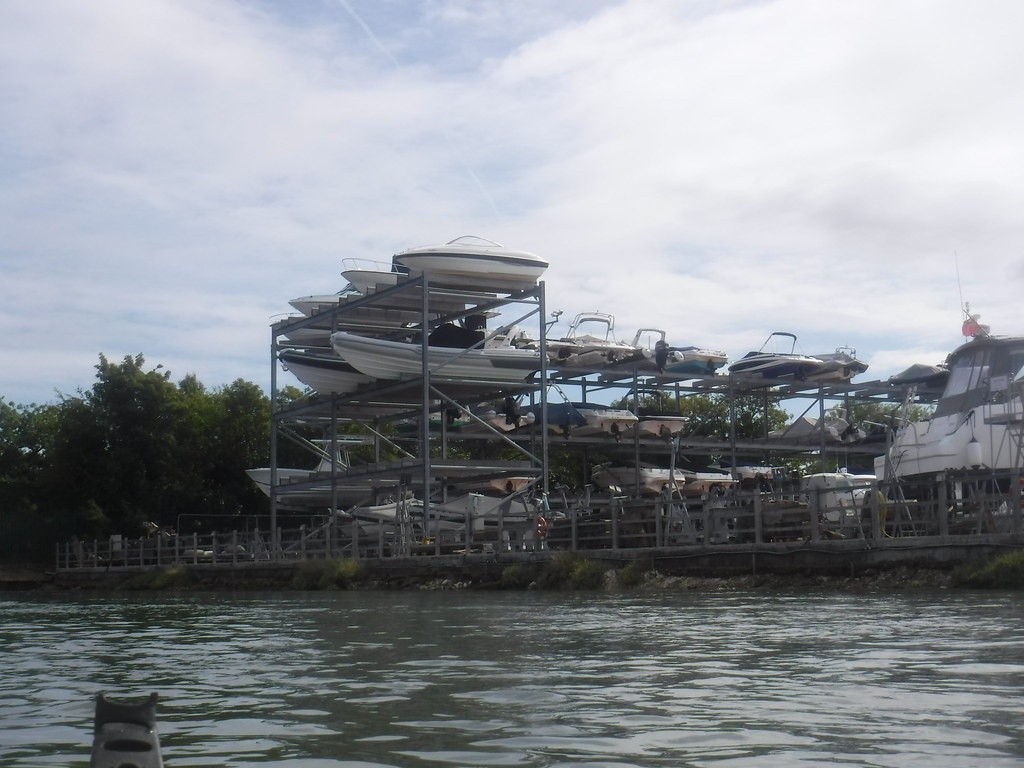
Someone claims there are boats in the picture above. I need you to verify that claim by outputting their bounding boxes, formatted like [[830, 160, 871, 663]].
[[245, 236, 1024, 537]]
